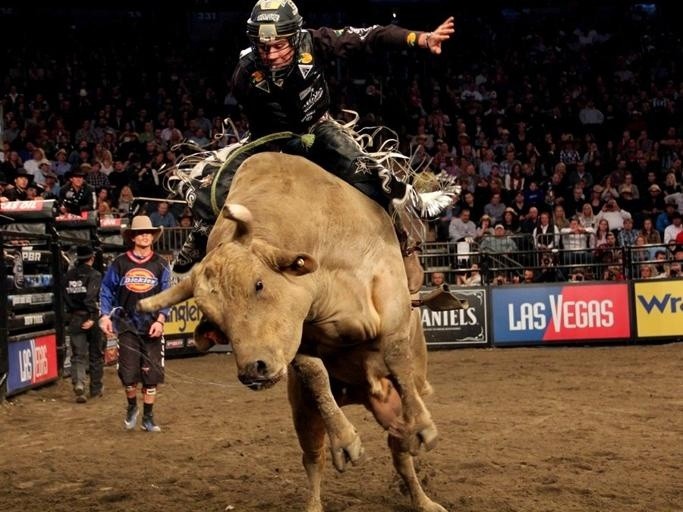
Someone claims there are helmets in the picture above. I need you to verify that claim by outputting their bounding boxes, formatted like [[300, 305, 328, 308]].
[[246, 0, 302, 48]]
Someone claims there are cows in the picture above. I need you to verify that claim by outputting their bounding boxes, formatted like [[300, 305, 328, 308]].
[[135, 151, 448, 512]]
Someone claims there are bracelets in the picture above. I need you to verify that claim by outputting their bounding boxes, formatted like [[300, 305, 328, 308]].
[[155, 319, 164, 326]]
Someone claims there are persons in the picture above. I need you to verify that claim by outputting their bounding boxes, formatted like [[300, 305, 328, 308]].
[[61, 240, 106, 407], [97, 213, 175, 432]]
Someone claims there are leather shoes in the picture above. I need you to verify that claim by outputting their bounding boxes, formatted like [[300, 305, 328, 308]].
[[403, 253, 424, 294]]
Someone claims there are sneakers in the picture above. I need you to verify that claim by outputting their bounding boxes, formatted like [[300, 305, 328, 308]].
[[124, 404, 139, 430], [73, 382, 87, 405], [140, 417, 161, 432], [90, 383, 105, 399]]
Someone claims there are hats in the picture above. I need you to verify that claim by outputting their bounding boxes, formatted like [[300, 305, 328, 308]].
[[123, 215, 163, 245], [416, 75, 661, 229], [11, 128, 140, 186], [75, 245, 96, 260]]
